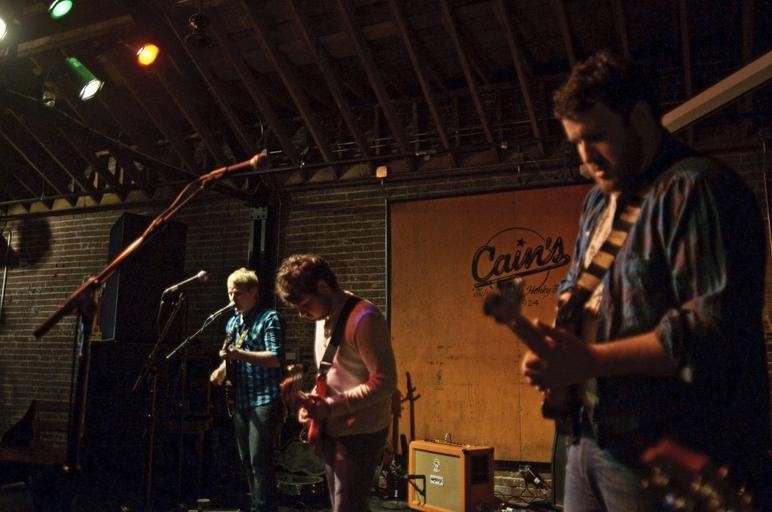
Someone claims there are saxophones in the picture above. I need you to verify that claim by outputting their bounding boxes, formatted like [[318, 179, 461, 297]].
[[226, 306, 239, 418]]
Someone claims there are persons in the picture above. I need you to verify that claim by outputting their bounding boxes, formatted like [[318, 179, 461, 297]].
[[206, 265, 291, 512], [516, 48, 770, 512], [272, 250, 400, 511]]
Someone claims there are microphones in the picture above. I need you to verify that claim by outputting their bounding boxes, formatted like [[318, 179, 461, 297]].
[[209, 300, 238, 318], [165, 270, 207, 292], [197, 154, 270, 184]]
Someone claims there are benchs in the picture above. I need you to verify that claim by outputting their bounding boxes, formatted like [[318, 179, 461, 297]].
[[0, 398, 69, 475]]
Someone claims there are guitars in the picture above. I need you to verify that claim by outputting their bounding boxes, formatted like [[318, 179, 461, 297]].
[[280, 365, 327, 455], [484, 276, 593, 436]]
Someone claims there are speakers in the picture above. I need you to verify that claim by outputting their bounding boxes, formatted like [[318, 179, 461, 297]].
[[406, 441, 494, 512]]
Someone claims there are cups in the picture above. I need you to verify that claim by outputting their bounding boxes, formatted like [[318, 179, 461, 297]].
[[196, 499, 210, 511]]
